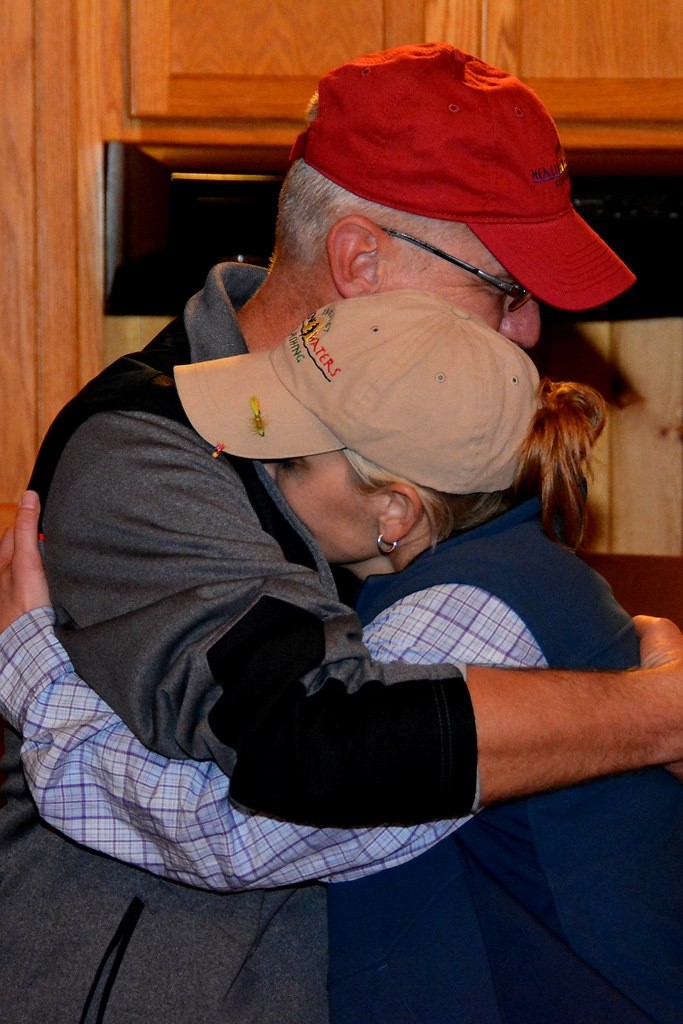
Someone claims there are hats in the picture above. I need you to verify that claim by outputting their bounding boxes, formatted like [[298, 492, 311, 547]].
[[288, 42, 638, 311], [173, 287, 541, 494]]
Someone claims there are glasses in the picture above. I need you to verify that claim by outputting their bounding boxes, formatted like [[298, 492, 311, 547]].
[[382, 226, 532, 312]]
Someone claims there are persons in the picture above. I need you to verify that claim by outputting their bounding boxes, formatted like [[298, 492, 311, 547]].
[[0, 41, 683, 1024]]
